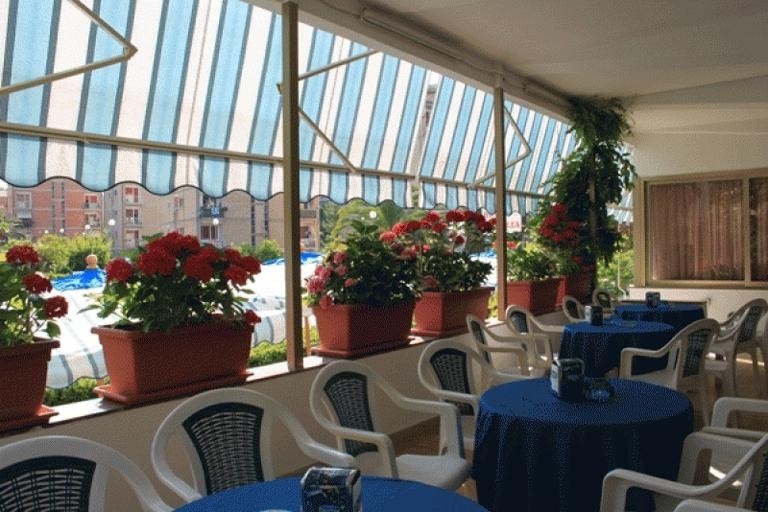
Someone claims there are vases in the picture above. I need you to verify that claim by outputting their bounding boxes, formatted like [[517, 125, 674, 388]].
[[565, 268, 597, 304]]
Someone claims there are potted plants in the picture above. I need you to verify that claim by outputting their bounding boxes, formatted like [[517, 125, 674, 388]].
[[506, 243, 562, 316]]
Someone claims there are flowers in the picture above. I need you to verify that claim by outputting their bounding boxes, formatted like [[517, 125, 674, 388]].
[[391, 209, 497, 292], [77, 233, 261, 327], [0, 244, 68, 349], [524, 201, 596, 282], [303, 231, 423, 309]]
